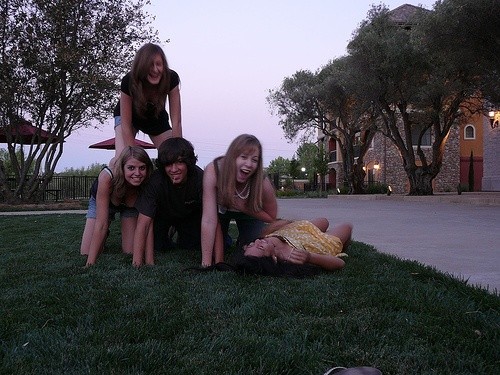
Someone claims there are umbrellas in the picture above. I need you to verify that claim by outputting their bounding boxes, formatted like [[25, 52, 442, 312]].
[[89, 138, 157, 149], [0, 119, 66, 146]]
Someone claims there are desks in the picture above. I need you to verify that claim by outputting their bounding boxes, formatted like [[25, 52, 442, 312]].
[[42, 189, 61, 202]]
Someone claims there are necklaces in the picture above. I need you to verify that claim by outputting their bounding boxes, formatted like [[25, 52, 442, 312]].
[[233, 179, 251, 199]]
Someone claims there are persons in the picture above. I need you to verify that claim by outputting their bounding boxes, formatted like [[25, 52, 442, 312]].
[[131, 137, 224, 270], [80, 146, 156, 268], [107, 43, 181, 167], [206, 216, 353, 279], [200, 133, 277, 270]]
